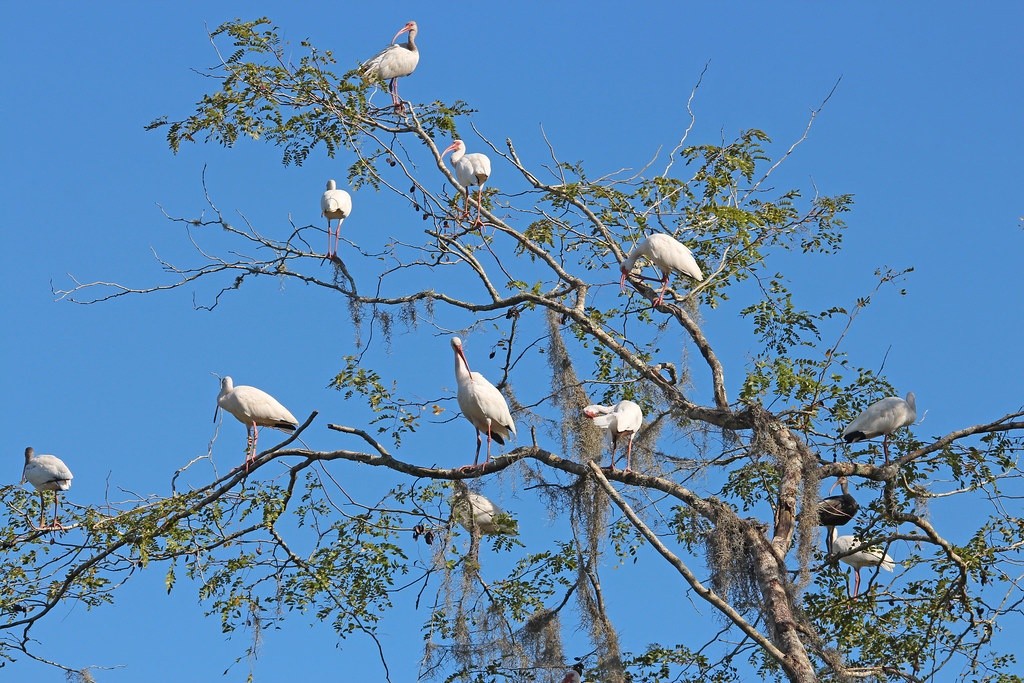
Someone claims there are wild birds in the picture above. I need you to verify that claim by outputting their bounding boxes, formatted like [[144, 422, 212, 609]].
[[795, 476, 898, 602], [619, 232, 707, 312], [18, 444, 76, 529], [211, 366, 300, 481], [444, 478, 515, 562], [319, 177, 353, 266], [836, 392, 920, 466], [451, 334, 520, 475], [353, 20, 420, 117], [441, 138, 494, 235], [581, 399, 644, 477]]
